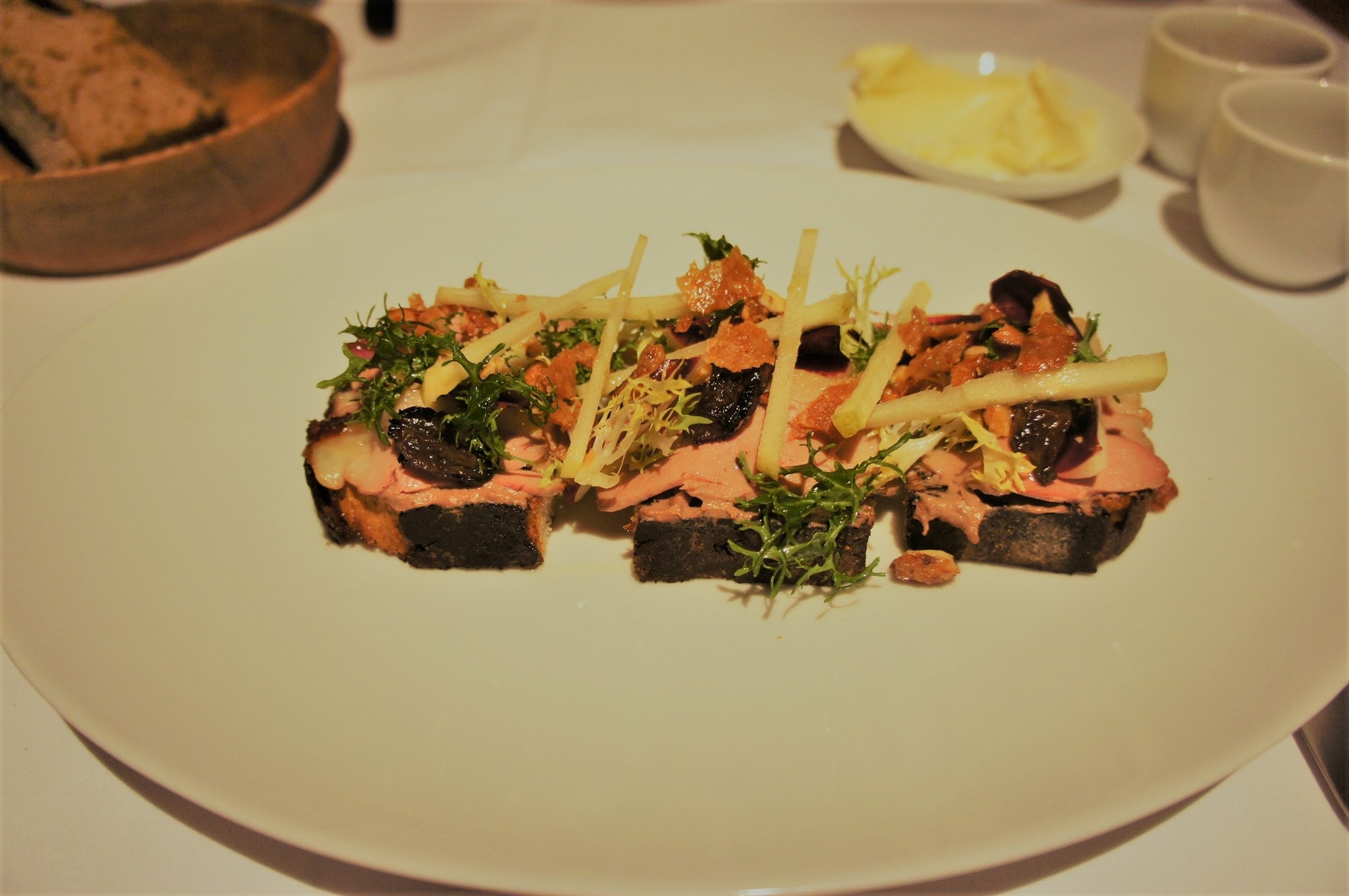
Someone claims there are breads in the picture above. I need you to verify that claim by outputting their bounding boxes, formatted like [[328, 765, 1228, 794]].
[[300, 368, 1154, 588], [0, 0, 227, 171]]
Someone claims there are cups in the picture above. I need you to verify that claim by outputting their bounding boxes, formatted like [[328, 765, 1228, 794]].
[[1143, 6, 1340, 174], [1194, 76, 1347, 289]]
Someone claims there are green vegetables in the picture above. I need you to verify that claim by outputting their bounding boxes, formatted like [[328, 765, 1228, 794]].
[[317, 232, 1113, 609]]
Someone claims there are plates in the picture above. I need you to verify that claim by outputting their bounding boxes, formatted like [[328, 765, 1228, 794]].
[[0, 1, 1348, 891], [840, 45, 1152, 201]]
